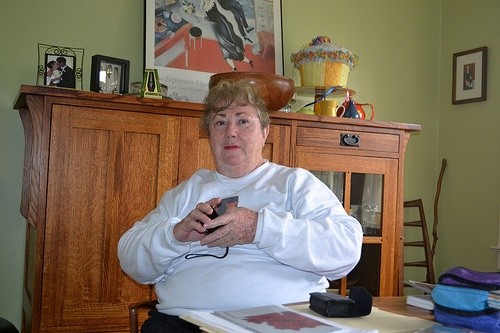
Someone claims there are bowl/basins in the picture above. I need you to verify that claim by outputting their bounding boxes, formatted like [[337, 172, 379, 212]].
[[209, 72, 295, 113], [130, 82, 169, 96]]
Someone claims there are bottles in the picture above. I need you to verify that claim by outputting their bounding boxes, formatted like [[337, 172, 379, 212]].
[[344, 100, 360, 119]]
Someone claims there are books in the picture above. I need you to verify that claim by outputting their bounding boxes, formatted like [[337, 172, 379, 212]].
[[406, 280, 435, 310]]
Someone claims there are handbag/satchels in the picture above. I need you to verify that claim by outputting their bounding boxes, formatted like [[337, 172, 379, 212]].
[[430, 266, 500, 333]]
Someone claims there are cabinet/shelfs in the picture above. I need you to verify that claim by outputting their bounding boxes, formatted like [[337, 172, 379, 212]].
[[12, 84, 422, 333]]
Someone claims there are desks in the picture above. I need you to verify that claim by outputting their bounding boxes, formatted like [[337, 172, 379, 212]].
[[284, 297, 437, 322]]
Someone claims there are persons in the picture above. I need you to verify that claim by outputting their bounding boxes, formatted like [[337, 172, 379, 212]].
[[117, 79, 363, 333], [46, 57, 75, 88]]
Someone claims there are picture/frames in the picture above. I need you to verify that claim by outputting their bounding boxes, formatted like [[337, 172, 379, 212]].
[[144, 0, 284, 104], [37, 42, 86, 91], [451, 47, 488, 103], [90, 55, 130, 95]]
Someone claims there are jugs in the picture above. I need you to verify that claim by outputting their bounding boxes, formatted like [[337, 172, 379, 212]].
[[336, 96, 375, 121]]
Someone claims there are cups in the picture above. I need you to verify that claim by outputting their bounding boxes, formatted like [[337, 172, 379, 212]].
[[319, 99, 345, 117]]
